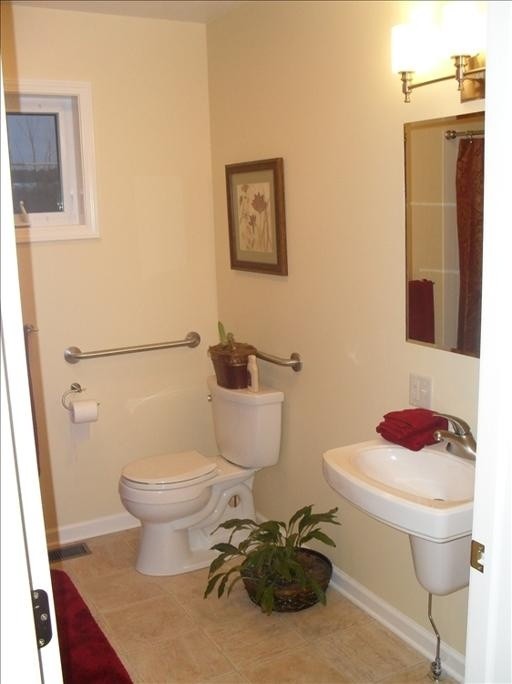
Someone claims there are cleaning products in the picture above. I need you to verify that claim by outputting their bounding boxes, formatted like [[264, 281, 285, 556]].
[[246, 354, 259, 391]]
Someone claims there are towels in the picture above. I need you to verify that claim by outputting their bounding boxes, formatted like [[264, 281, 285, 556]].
[[377, 405, 447, 450]]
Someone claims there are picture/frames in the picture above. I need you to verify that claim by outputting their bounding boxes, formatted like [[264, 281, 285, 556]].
[[224, 157, 288, 276], [2, 77, 102, 243]]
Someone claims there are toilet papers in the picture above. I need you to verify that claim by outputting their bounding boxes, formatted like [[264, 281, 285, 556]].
[[69, 399, 98, 423]]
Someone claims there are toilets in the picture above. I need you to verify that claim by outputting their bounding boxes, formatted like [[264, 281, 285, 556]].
[[118, 374, 284, 577]]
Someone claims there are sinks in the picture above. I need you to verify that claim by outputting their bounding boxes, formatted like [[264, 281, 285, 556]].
[[322, 439, 476, 543]]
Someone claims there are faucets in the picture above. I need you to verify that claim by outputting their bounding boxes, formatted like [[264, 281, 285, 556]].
[[432, 413, 476, 460]]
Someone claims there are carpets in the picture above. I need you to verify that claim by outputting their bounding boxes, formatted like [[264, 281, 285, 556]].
[[51, 566, 134, 683]]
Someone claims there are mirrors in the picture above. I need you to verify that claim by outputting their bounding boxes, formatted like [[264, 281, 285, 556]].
[[401, 112, 481, 361]]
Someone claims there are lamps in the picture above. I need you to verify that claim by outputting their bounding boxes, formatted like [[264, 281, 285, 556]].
[[389, 8, 486, 102]]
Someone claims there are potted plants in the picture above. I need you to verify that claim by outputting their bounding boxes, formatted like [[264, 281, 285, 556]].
[[201, 503, 345, 622]]
